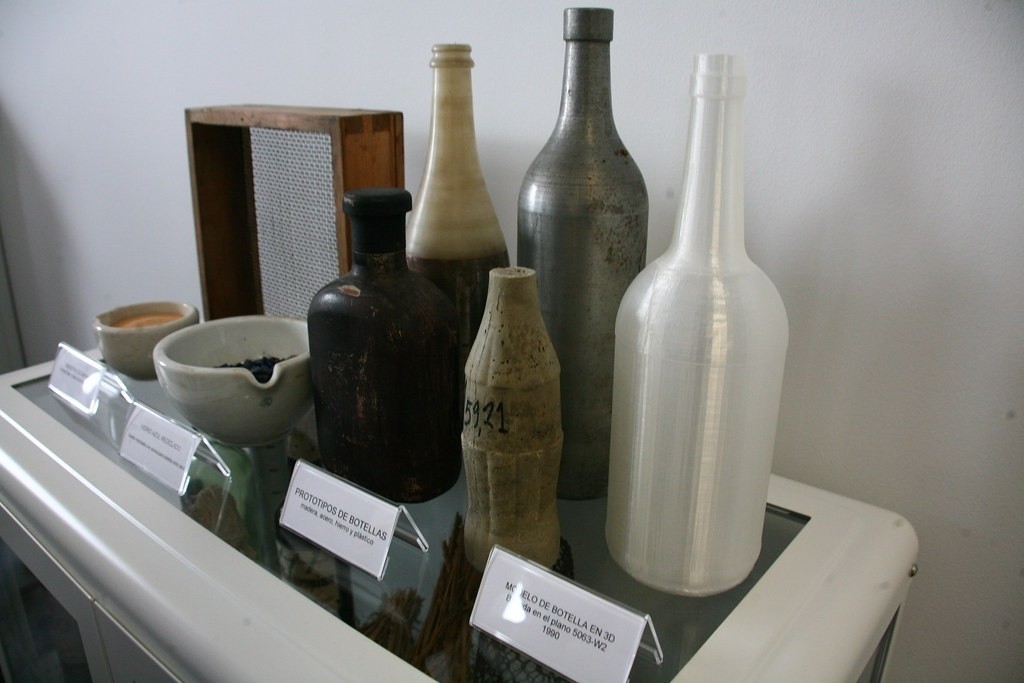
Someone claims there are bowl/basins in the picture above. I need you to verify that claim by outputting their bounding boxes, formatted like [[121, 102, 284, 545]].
[[152, 315, 310, 445], [93, 299, 199, 381]]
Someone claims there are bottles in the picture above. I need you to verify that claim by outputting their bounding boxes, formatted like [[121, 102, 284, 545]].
[[405, 41, 510, 384], [459, 268, 560, 575], [516, 5, 648, 496], [608, 51, 791, 598], [307, 187, 459, 507]]
